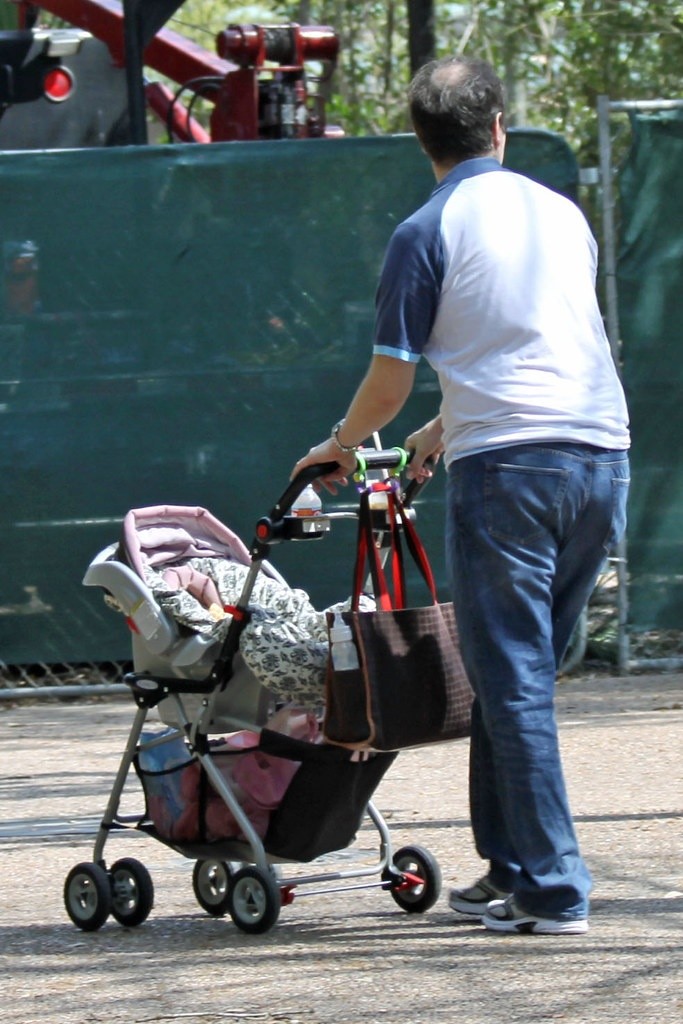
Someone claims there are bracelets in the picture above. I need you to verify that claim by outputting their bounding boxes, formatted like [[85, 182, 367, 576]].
[[331, 418, 357, 452]]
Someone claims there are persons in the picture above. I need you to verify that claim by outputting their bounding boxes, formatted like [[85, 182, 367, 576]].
[[290, 54, 632, 934]]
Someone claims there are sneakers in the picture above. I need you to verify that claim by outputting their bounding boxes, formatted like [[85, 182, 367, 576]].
[[483, 894, 588, 935], [447, 872, 511, 914]]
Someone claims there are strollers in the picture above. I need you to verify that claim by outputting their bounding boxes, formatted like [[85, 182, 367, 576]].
[[65, 444, 442, 931]]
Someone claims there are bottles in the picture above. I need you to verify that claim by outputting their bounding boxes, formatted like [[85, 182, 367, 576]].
[[330, 605, 360, 670], [290, 483, 322, 540]]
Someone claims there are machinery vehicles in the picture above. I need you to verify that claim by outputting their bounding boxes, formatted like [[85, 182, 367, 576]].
[[0, 0, 347, 152]]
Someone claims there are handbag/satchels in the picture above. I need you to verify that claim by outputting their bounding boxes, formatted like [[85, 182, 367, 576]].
[[325, 488, 472, 754]]
